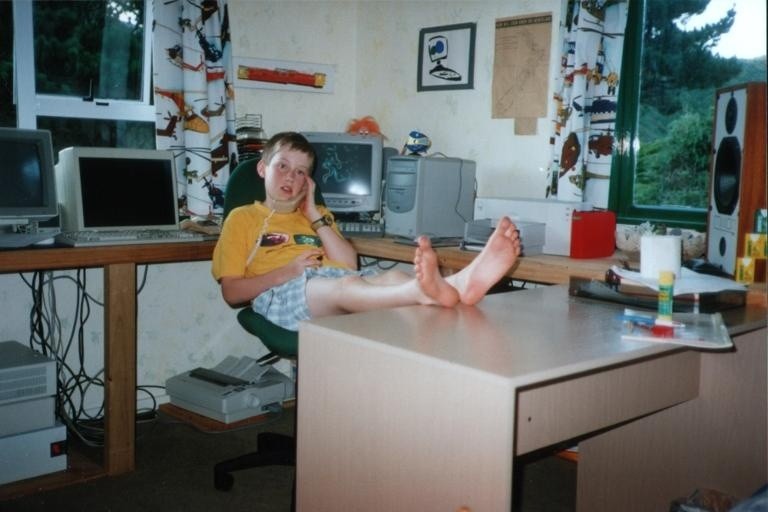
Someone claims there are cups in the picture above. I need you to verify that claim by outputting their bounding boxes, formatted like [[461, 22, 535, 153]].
[[641, 235, 682, 283]]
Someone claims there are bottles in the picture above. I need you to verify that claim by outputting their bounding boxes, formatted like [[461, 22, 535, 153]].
[[658, 271, 673, 323]]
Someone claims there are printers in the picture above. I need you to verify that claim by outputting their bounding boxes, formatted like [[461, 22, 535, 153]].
[[165, 355, 287, 424]]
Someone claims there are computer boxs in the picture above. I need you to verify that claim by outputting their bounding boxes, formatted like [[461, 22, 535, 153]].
[[385, 156, 475, 247]]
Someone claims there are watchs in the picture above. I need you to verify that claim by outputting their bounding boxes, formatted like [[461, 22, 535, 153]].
[[311, 214, 333, 232]]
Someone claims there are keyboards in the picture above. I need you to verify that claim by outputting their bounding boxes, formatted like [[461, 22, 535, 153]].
[[57, 230, 204, 247], [335, 220, 384, 238]]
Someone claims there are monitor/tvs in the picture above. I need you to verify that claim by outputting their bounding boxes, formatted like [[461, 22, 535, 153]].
[[0, 126, 60, 233], [300, 132, 383, 222], [55, 147, 180, 232]]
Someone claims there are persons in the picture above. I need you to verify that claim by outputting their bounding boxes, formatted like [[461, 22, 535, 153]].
[[211, 131, 524, 332]]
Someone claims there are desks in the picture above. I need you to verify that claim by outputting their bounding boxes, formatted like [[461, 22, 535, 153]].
[[0, 232, 382, 502], [294, 283, 768, 512], [350, 236, 768, 308]]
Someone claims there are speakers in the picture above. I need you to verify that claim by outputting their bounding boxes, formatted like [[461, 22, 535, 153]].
[[705, 82, 768, 279]]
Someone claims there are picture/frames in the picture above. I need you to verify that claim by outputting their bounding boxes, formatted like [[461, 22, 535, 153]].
[[416, 22, 476, 92]]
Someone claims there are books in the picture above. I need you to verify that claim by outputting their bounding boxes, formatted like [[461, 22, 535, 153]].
[[621, 307, 736, 350]]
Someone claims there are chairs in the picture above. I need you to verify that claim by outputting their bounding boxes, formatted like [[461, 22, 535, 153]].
[[213, 157, 360, 492]]
